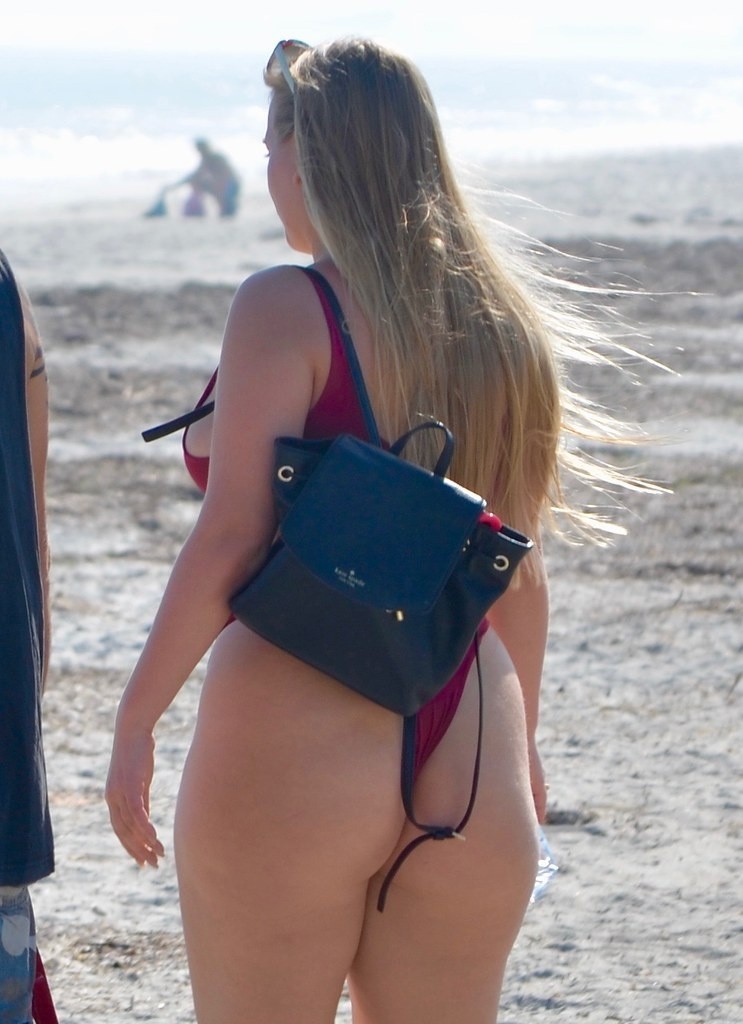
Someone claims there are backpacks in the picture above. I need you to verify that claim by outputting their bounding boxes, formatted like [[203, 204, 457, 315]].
[[142, 269, 536, 914]]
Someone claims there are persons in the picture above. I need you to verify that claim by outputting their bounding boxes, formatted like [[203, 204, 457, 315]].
[[0, 248, 56, 1024], [188, 136, 240, 217], [103, 38, 563, 1024]]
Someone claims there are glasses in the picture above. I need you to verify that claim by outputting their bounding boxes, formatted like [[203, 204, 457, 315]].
[[267, 39, 311, 99]]
